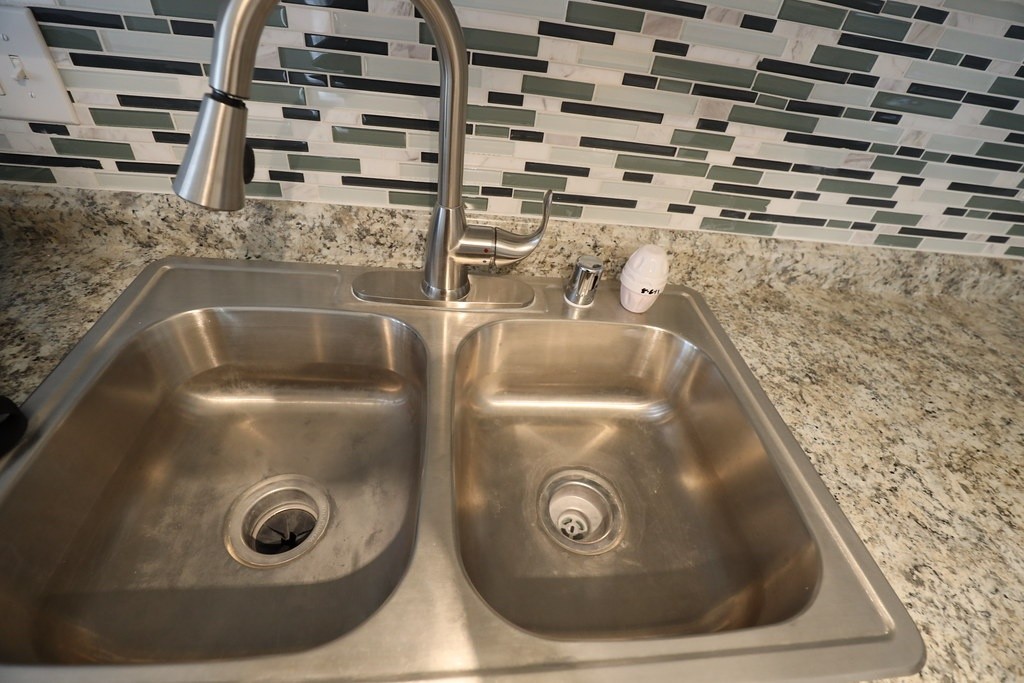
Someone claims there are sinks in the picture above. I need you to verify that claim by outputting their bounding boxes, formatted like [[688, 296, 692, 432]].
[[441, 264, 928, 683], [0, 253, 438, 683]]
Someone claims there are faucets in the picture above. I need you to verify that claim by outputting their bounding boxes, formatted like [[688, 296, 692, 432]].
[[173, 0, 466, 300]]
